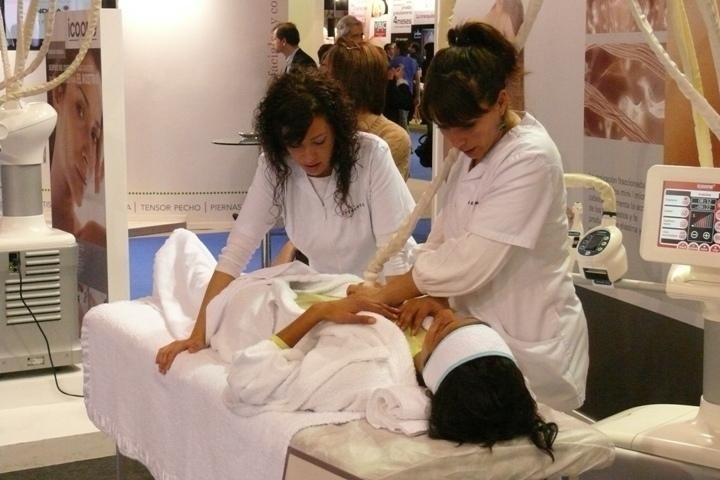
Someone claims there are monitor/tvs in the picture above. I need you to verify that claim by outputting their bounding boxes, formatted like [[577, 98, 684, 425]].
[[639, 164, 720, 268]]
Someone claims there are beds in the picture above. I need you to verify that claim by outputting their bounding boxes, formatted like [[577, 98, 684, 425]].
[[80, 297, 617, 480]]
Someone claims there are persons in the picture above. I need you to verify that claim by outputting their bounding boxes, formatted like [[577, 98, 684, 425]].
[[348, 21, 589, 413], [44, 48, 107, 336], [324, 44, 411, 186], [154, 68, 421, 374], [152, 229, 558, 463], [271, 23, 318, 76], [334, 15, 363, 47], [374, 43, 434, 127]]
